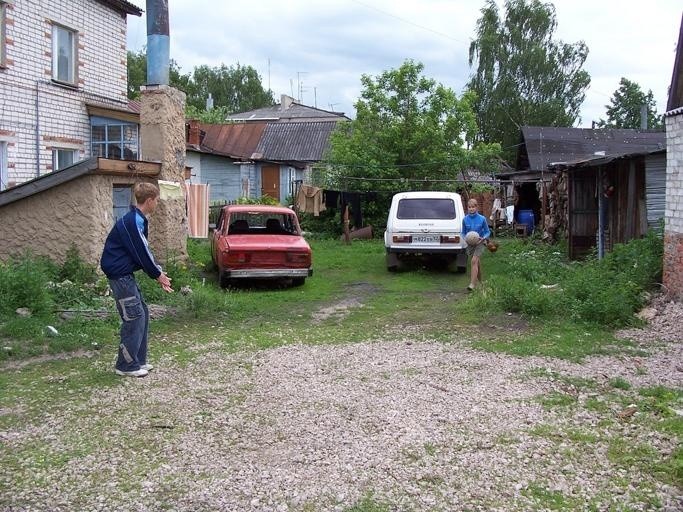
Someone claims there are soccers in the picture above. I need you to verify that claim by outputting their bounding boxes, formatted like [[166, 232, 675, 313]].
[[466, 231, 480, 245]]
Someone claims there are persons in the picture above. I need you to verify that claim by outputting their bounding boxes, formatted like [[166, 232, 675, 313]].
[[461, 196, 490, 291], [99, 180, 174, 378]]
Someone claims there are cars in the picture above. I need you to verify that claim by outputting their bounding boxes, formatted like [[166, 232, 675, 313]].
[[211, 204, 312, 290]]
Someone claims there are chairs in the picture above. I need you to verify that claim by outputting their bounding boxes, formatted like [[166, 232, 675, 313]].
[[231, 220, 250, 233], [265, 218, 283, 234]]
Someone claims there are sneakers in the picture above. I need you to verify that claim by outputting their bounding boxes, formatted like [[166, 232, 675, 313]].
[[116, 368, 149, 377], [467, 285, 477, 290], [138, 363, 153, 371]]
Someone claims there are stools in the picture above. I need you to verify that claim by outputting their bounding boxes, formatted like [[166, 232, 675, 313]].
[[514, 224, 529, 238]]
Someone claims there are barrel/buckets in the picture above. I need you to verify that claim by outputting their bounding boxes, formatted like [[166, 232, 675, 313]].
[[517, 209, 534, 235]]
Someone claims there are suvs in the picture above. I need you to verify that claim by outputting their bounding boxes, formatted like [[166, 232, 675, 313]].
[[384, 191, 468, 273]]
